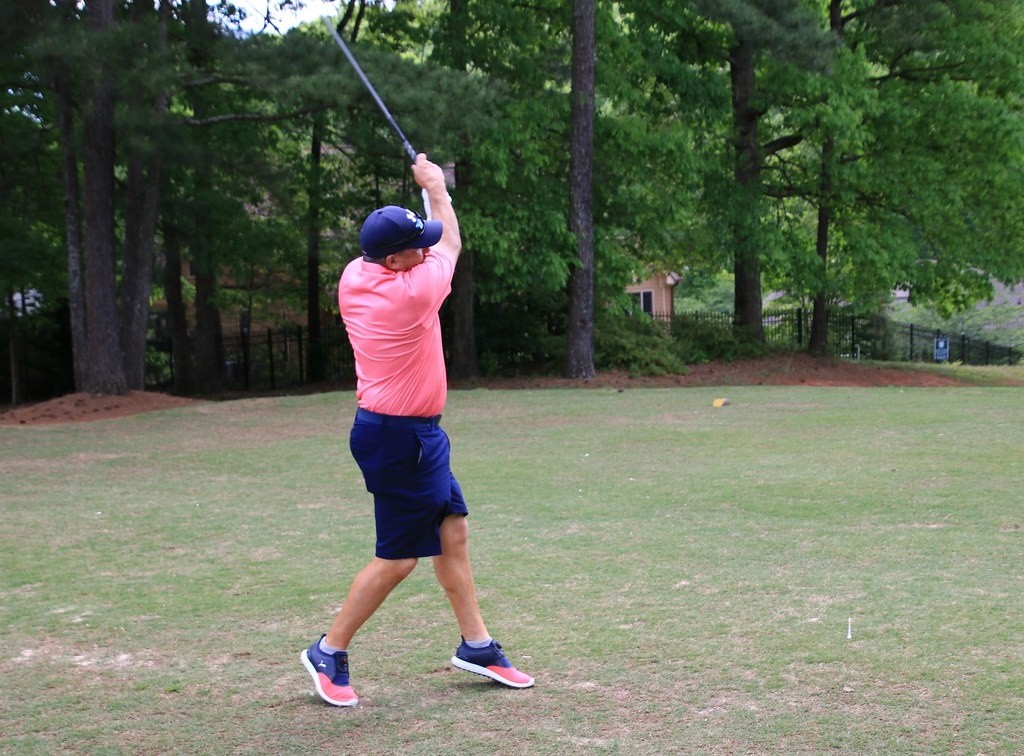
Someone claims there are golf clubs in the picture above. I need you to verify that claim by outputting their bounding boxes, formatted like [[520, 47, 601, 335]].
[[319, 12, 418, 165]]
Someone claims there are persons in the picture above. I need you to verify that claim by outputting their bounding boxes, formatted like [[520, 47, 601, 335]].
[[297, 151, 535, 710]]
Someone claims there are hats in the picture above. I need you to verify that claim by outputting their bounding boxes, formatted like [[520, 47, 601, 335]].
[[359, 206, 443, 258]]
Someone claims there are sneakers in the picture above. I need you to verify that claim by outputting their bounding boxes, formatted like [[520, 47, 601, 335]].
[[451, 634, 534, 688], [300, 633, 359, 706]]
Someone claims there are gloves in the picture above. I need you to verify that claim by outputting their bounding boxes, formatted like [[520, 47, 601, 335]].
[[422, 186, 452, 220]]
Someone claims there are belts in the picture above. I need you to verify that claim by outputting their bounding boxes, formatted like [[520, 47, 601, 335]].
[[356, 408, 442, 427]]
